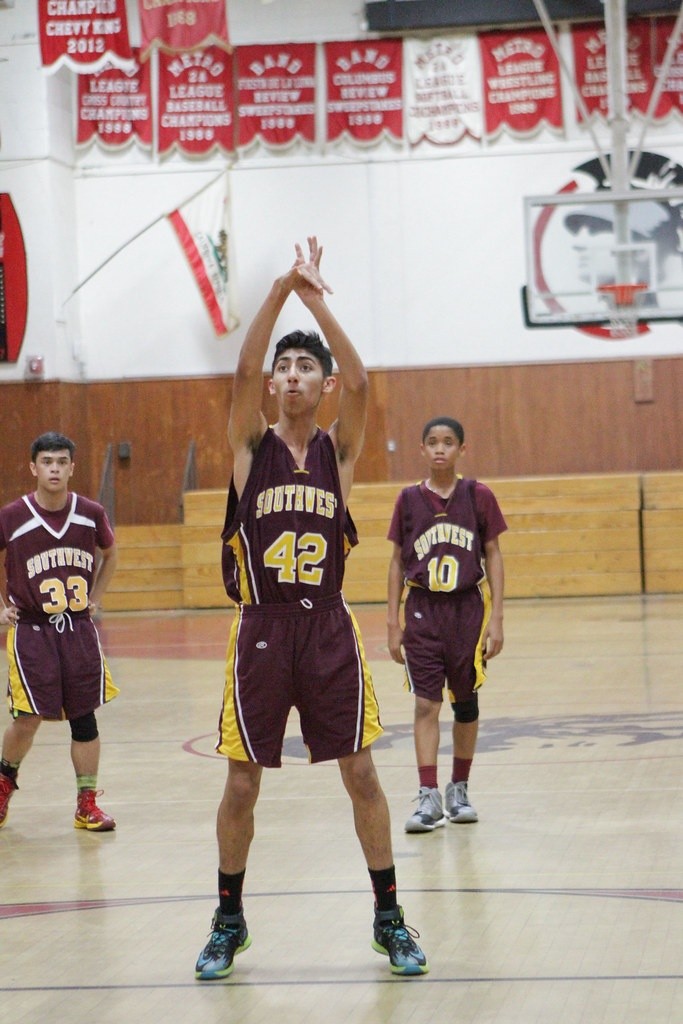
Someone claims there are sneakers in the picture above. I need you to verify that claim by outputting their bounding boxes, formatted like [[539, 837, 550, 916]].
[[74, 790, 116, 830], [443, 781, 478, 822], [372, 902, 429, 975], [0, 772, 19, 828], [405, 787, 447, 831], [195, 906, 251, 978]]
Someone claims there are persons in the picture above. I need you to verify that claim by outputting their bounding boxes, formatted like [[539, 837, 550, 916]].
[[1, 431, 117, 832], [193, 237, 430, 976], [385, 417, 508, 834]]
